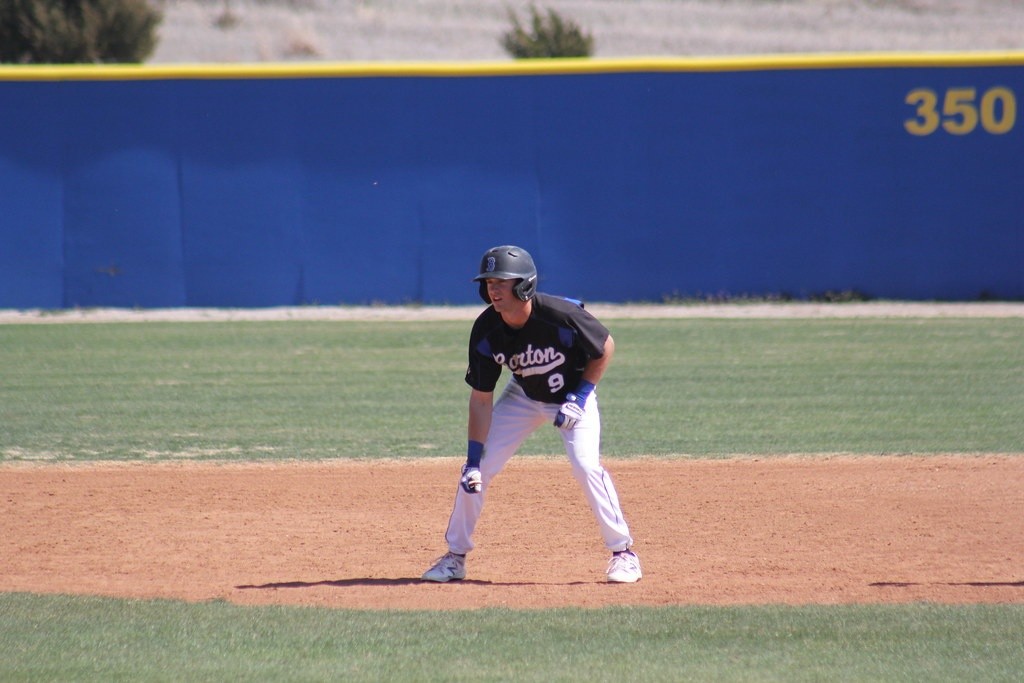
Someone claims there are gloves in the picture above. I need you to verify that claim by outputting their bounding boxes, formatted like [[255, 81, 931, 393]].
[[553, 378, 595, 431], [460, 438, 485, 494]]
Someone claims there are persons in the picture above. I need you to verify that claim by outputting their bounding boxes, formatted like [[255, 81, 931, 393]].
[[419, 244, 641, 584]]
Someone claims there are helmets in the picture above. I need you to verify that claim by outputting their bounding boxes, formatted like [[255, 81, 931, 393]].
[[471, 245, 537, 304]]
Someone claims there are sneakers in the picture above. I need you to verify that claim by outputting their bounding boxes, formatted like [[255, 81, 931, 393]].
[[421, 553, 466, 583], [605, 551, 642, 583]]
